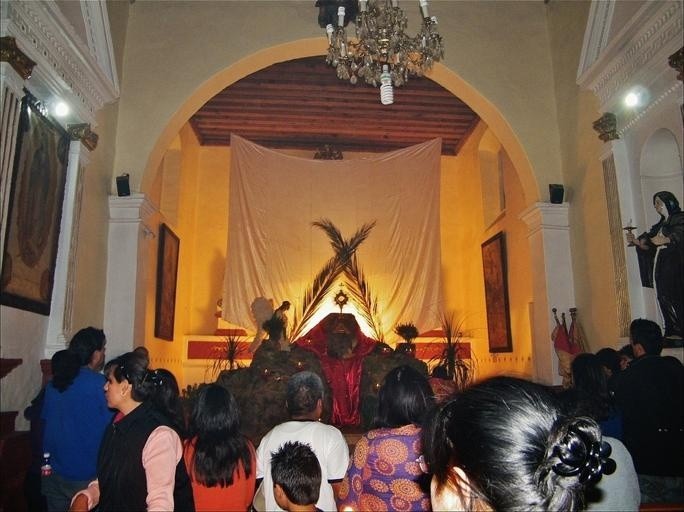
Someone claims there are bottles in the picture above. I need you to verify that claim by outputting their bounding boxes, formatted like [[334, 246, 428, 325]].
[[41, 452, 52, 476]]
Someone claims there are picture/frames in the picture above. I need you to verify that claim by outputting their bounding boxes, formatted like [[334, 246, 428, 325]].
[[154, 223, 180, 341], [481, 229, 513, 353], [0, 85, 70, 316]]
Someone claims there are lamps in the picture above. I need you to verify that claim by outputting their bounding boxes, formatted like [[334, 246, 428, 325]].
[[324, 0, 445, 106]]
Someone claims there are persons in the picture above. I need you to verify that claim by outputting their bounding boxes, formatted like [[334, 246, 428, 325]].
[[626, 191, 684, 345], [41, 326, 349, 512], [269, 301, 291, 346], [336, 320, 683, 512]]
